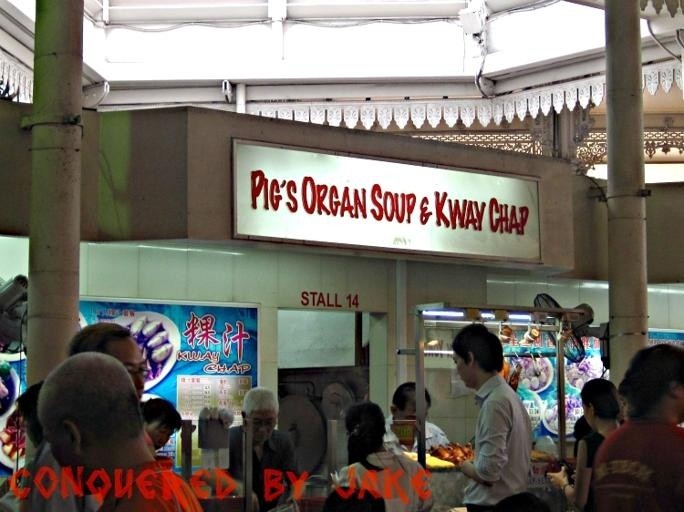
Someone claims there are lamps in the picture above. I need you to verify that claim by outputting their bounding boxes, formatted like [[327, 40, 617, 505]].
[[425, 328, 439, 346]]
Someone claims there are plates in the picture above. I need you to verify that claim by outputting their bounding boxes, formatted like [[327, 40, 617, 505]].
[[528, 355, 594, 436], [135, 311, 183, 393], [1, 352, 27, 471]]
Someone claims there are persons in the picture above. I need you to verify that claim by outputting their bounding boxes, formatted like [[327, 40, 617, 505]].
[[329, 401, 435, 512], [381, 380, 448, 454], [204, 386, 298, 510], [546, 340, 683, 512], [0, 380, 77, 512], [34, 352, 205, 511], [141, 398, 182, 454], [67, 320, 143, 402], [452, 324, 532, 511]]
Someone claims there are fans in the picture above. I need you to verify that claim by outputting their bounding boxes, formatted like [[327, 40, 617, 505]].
[[534, 293, 610, 369]]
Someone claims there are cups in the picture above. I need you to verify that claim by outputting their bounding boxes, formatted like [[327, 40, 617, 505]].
[[531, 462, 549, 480]]
